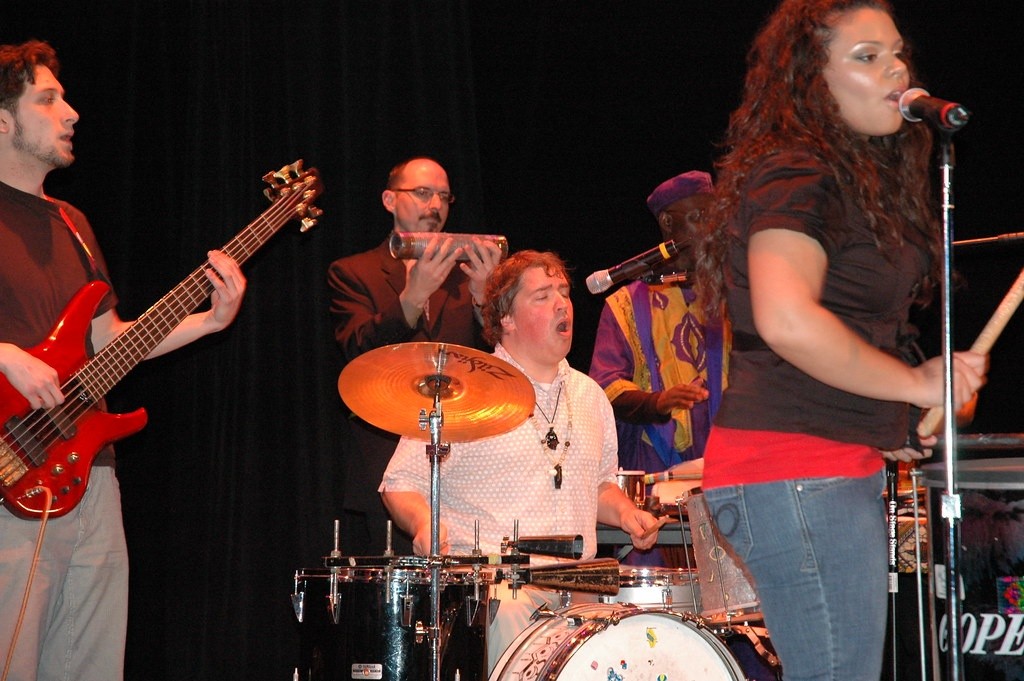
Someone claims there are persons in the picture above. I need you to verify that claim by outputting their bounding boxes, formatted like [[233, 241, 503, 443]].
[[686, 0, 994, 681], [378, 248, 658, 564], [328, 155, 510, 568], [0, 40, 250, 681], [590, 169, 783, 681]]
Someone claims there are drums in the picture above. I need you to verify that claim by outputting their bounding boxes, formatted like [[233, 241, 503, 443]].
[[596, 564, 704, 618], [674, 486, 764, 626], [487, 600, 752, 681], [287, 553, 502, 681], [921, 458, 1024, 681], [714, 624, 784, 681]]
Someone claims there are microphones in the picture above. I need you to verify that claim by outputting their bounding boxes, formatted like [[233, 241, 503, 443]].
[[586, 239, 685, 295], [899, 87, 971, 129]]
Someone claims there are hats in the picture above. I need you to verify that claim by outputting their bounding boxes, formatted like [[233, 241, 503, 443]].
[[647, 170, 716, 220]]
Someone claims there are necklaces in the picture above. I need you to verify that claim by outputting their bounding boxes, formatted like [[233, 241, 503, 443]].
[[528, 380, 574, 490]]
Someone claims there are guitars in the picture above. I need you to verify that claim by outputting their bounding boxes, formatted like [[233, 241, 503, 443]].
[[0, 157, 329, 523]]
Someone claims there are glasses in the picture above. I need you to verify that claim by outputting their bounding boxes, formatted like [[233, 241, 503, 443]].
[[390, 186, 455, 204], [666, 207, 705, 225]]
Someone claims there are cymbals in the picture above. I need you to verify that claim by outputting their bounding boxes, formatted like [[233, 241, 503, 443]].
[[337, 341, 535, 445]]
